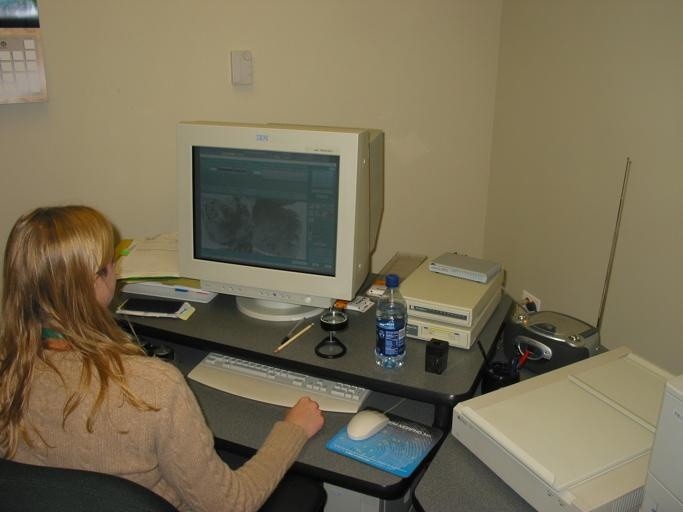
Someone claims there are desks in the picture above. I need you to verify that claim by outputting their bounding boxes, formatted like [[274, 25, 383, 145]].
[[108, 270, 537, 510]]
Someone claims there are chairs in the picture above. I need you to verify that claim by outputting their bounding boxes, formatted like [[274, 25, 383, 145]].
[[1, 455, 328, 512]]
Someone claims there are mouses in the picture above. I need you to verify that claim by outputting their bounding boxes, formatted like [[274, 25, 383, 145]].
[[347, 409, 389, 440]]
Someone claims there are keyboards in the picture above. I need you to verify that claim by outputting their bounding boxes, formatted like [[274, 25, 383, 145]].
[[187, 352, 374, 413]]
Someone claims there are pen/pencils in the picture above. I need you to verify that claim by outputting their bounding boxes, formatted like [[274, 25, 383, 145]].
[[274, 317, 315, 353], [477, 341, 529, 373]]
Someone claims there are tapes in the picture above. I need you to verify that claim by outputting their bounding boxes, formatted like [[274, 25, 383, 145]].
[[149, 347, 175, 360]]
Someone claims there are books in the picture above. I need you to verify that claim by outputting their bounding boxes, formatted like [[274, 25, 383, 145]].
[[116, 272, 218, 304]]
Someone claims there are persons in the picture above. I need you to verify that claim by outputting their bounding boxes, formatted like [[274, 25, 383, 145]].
[[0, 203, 330, 511]]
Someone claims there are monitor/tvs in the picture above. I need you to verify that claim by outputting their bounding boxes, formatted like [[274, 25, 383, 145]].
[[176, 120, 384, 322]]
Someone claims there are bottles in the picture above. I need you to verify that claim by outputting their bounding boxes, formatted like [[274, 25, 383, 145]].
[[480, 361, 519, 395], [376, 273, 408, 367]]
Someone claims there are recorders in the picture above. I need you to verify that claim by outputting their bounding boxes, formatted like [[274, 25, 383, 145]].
[[503, 311, 600, 375]]
